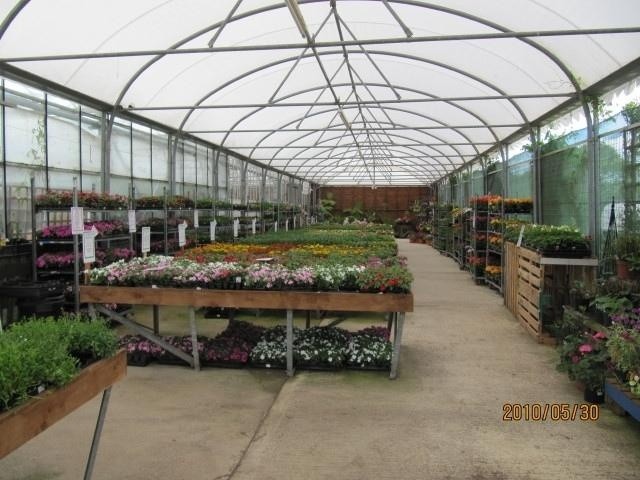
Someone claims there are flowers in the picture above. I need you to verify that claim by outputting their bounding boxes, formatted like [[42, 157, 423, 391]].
[[121, 323, 392, 370], [573, 312, 638, 364], [88, 243, 413, 291]]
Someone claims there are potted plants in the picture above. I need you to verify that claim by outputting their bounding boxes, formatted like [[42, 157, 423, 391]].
[[0, 310, 127, 459]]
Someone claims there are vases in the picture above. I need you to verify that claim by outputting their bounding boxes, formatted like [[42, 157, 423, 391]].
[[615, 258, 634, 279], [584, 381, 604, 404]]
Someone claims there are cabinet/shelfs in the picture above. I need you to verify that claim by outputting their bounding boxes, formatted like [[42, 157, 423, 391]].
[[503, 239, 599, 345], [35, 190, 317, 322], [77, 286, 416, 378], [412, 197, 533, 296]]
